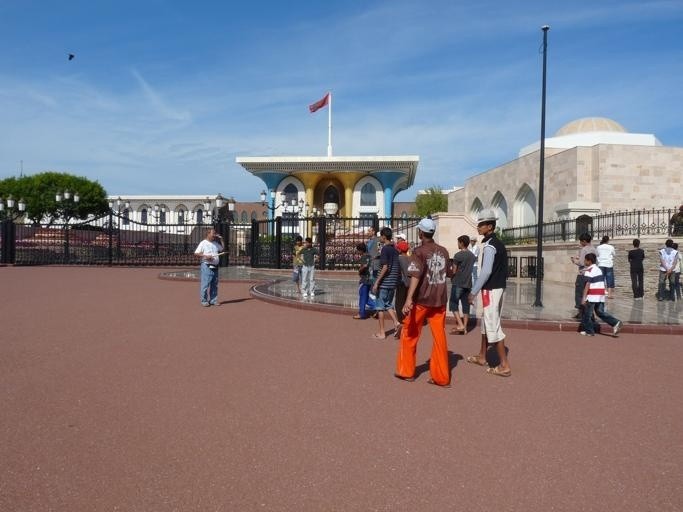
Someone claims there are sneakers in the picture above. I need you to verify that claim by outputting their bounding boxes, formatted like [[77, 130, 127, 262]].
[[613, 320, 623, 335]]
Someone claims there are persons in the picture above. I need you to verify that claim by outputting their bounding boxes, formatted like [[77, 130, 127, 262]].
[[570, 233, 623, 338], [658, 239, 682, 302], [292, 236, 319, 297], [628, 238, 645, 299], [394, 219, 452, 385], [467, 210, 512, 377], [194, 229, 225, 307], [450, 235, 480, 338], [355, 226, 412, 342]]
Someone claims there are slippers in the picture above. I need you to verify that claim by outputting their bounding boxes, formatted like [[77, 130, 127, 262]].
[[487, 367, 511, 377], [451, 326, 468, 335], [467, 355, 489, 366]]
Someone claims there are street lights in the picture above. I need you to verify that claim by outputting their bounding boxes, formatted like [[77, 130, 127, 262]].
[[176, 206, 194, 254], [55, 188, 79, 262], [0, 194, 26, 264], [259, 187, 286, 259], [106, 196, 130, 261], [147, 201, 166, 257], [280, 195, 327, 246], [203, 193, 235, 266]]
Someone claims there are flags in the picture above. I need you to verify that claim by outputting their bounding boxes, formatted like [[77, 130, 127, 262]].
[[309, 94, 328, 112]]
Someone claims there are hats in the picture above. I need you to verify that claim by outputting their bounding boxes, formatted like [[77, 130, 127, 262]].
[[416, 218, 435, 233], [476, 209, 498, 224], [395, 233, 406, 241]]
